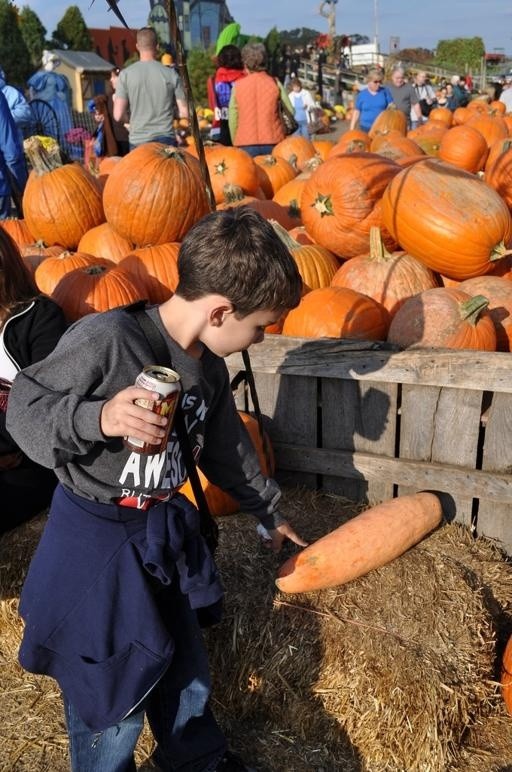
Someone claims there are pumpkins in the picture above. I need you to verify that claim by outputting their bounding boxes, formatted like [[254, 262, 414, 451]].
[[502, 636, 512, 721], [3, 99, 512, 351], [181, 412, 275, 516], [273, 491, 443, 595]]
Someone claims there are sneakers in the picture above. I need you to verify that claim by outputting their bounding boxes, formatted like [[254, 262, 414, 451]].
[[150, 744, 257, 772]]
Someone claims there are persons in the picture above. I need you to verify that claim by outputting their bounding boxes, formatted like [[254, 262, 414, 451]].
[[6, 208, 310, 765], [1, 28, 512, 219], [0, 226, 68, 535]]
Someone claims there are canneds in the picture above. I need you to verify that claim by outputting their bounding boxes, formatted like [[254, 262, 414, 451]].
[[124, 366, 182, 453]]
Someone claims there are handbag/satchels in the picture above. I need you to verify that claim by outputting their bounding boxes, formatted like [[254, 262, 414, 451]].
[[419, 99, 434, 117], [278, 99, 308, 135]]
